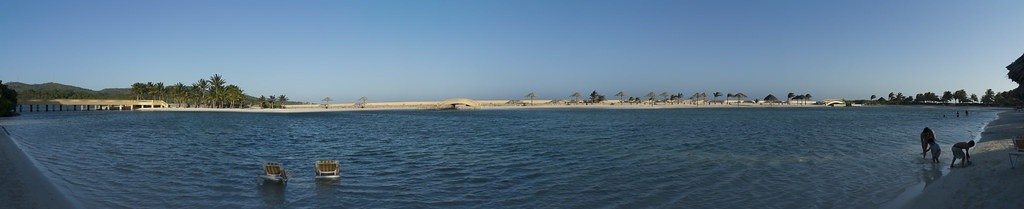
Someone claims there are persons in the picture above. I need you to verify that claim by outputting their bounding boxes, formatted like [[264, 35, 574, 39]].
[[921, 127, 941, 163], [965, 109, 968, 116], [956, 111, 959, 117], [950, 140, 975, 168]]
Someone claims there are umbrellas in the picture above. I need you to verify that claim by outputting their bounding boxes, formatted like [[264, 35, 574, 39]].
[[792, 94, 812, 103], [570, 92, 582, 103], [358, 96, 368, 103], [692, 91, 709, 104], [614, 91, 628, 101], [734, 93, 748, 102], [646, 91, 671, 105], [524, 92, 538, 104], [764, 94, 778, 105], [322, 97, 333, 103]]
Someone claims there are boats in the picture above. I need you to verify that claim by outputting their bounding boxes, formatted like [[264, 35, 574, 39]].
[[260, 161, 288, 183], [314, 159, 341, 178]]
